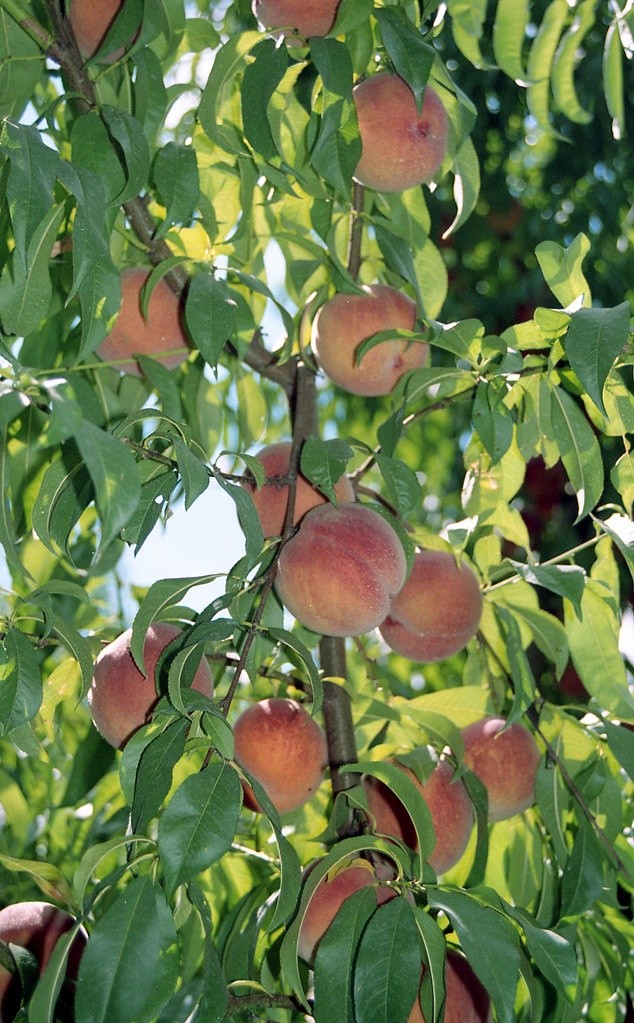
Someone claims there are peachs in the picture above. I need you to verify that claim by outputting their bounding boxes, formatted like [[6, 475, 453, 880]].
[[1, 0, 541, 1023]]
